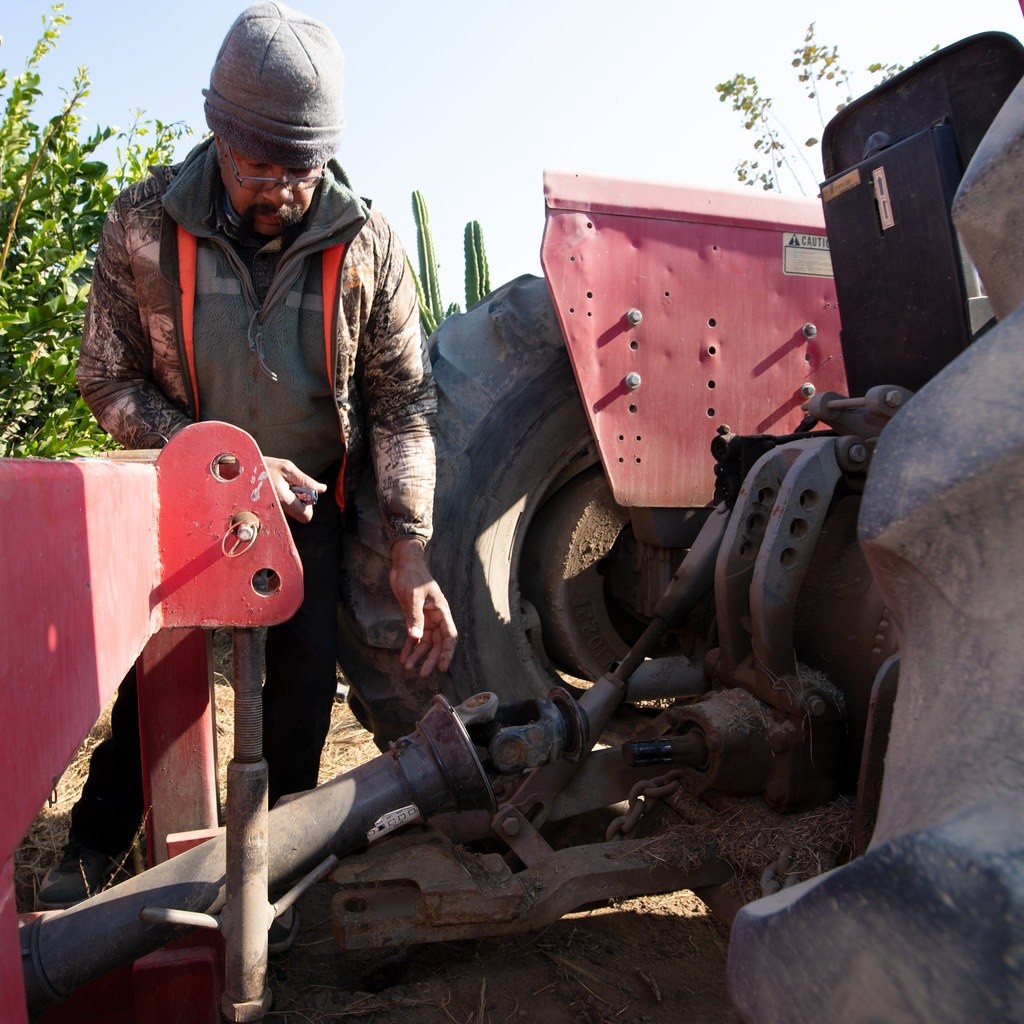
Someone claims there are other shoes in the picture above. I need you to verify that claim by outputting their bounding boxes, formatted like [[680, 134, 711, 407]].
[[36, 840, 116, 911]]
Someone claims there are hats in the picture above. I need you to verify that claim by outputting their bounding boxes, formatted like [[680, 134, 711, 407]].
[[201, 1, 345, 169]]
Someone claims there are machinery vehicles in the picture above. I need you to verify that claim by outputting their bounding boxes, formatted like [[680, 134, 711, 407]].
[[0, 32, 1024, 1024]]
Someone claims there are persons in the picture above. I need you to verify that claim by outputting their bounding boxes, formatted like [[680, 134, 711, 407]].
[[31, 1, 459, 913]]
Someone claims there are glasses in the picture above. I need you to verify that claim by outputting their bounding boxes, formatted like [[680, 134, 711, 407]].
[[227, 144, 327, 192]]
